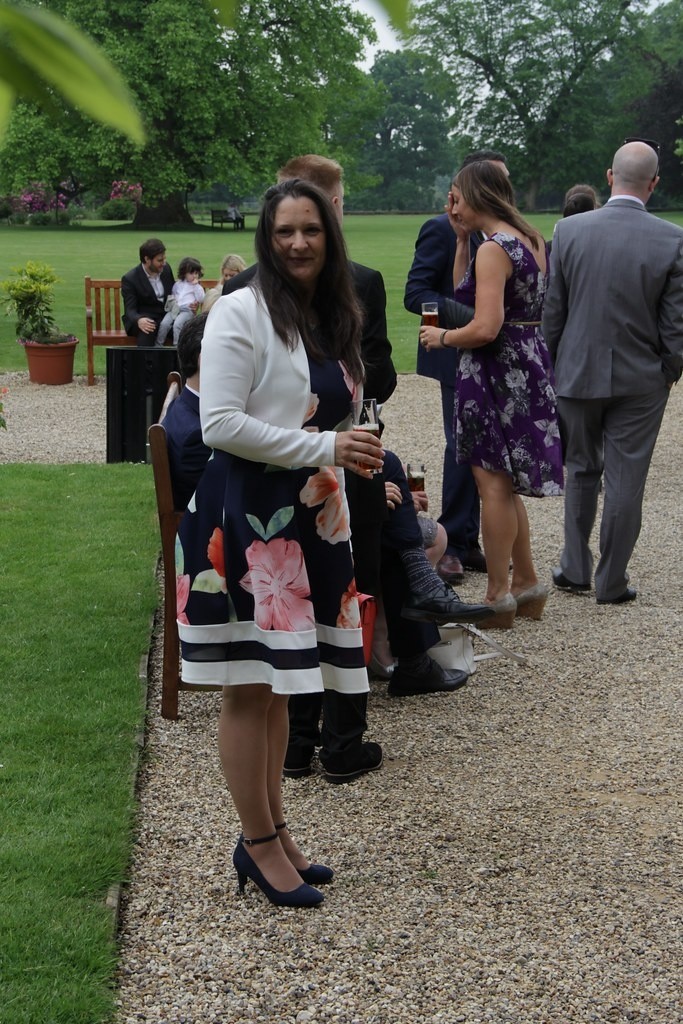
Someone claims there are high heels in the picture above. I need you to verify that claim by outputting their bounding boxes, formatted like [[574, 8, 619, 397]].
[[233, 833, 324, 908], [473, 591, 517, 628], [509, 584, 549, 620], [274, 823, 333, 884]]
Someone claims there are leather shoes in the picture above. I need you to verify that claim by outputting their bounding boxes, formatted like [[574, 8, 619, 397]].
[[550, 568, 590, 594], [597, 589, 637, 604], [386, 662, 468, 698], [462, 552, 513, 572], [437, 553, 464, 581], [406, 583, 496, 622]]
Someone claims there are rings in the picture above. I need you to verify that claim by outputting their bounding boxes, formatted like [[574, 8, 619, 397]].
[[422, 339, 427, 345]]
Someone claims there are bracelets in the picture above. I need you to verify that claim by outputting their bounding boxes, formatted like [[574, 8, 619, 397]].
[[439, 329, 450, 348]]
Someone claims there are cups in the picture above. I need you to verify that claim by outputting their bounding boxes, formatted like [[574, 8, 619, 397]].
[[421, 302, 439, 329], [351, 397, 382, 474], [407, 463, 425, 492]]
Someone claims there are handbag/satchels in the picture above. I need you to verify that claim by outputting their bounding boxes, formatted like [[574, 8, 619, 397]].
[[424, 621, 525, 676], [435, 299, 507, 357]]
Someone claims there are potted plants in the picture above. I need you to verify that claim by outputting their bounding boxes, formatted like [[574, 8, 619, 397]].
[[0, 260, 79, 385]]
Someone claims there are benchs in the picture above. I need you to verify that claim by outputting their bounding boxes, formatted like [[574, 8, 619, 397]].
[[84, 276, 219, 386], [210, 210, 245, 231], [148, 371, 224, 720]]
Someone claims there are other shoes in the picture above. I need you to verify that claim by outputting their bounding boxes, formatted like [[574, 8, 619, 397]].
[[319, 741, 382, 784], [279, 744, 315, 777]]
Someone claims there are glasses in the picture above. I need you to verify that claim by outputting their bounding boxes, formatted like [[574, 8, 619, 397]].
[[610, 137, 661, 182]]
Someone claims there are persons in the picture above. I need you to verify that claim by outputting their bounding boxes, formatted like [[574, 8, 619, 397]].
[[170, 179, 384, 911], [220, 151, 399, 784], [201, 252, 249, 312], [402, 149, 513, 570], [545, 183, 601, 255], [418, 162, 565, 632], [154, 257, 206, 347], [228, 202, 242, 233], [120, 238, 175, 347], [541, 140, 683, 605], [161, 310, 497, 699]]
